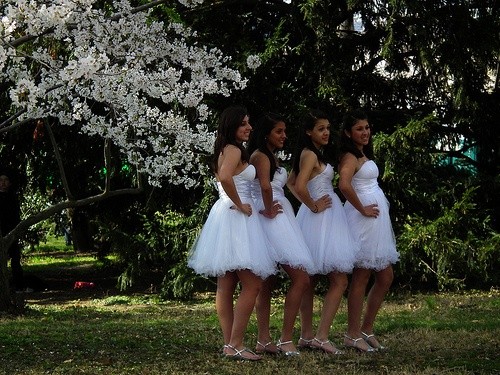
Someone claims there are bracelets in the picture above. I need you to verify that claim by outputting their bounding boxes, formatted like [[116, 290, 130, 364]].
[[312, 205, 319, 214]]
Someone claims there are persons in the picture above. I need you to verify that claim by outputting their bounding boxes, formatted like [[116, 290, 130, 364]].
[[286, 108, 357, 354], [339, 110, 400, 352], [247, 111, 317, 356], [187, 103, 276, 360]]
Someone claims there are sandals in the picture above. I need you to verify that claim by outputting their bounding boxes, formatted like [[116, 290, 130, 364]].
[[255, 339, 282, 354], [360, 331, 388, 349], [344, 335, 378, 352], [276, 339, 301, 356], [222, 344, 262, 360], [297, 337, 314, 347], [312, 337, 346, 355]]
